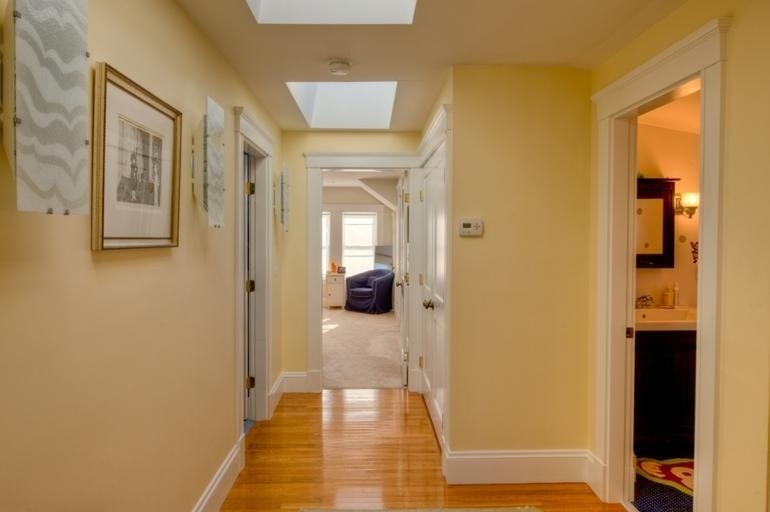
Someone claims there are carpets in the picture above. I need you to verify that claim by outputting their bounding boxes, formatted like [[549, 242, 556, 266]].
[[322, 308, 403, 389], [636, 458, 693, 497]]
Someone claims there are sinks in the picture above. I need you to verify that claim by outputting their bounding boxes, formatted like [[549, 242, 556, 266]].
[[635, 308, 697, 324]]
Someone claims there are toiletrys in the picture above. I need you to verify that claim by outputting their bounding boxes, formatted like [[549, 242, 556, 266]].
[[674, 281, 680, 305], [662, 283, 674, 305]]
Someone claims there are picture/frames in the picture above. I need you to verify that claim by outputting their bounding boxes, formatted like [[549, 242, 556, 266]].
[[91, 62, 182, 252]]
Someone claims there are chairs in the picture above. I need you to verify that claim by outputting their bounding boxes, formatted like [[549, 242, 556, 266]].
[[346, 269, 394, 314]]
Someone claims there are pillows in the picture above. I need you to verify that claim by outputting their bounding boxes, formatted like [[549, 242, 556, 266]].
[[367, 276, 378, 287]]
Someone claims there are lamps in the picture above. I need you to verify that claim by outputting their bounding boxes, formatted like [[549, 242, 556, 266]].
[[673, 192, 698, 219], [329, 59, 351, 76]]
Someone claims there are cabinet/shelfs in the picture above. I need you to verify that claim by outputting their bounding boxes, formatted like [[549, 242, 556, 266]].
[[326, 272, 346, 309]]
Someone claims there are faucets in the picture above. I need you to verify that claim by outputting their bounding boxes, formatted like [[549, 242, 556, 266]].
[[636, 294, 656, 307]]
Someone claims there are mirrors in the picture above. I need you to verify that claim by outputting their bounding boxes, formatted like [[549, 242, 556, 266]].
[[637, 182, 675, 268]]
